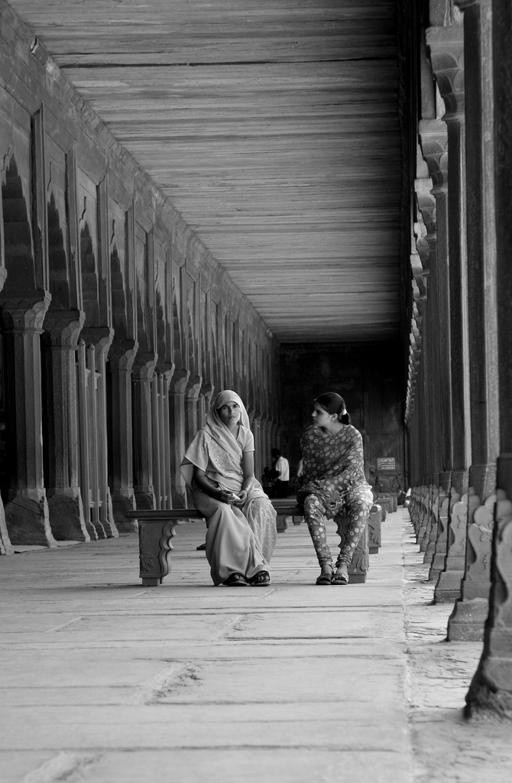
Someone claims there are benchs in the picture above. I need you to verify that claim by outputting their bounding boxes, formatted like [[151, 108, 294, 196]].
[[123, 490, 402, 589]]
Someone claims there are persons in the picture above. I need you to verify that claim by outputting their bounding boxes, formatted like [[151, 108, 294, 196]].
[[180, 389, 278, 585], [261, 447, 291, 498], [301, 391, 373, 582]]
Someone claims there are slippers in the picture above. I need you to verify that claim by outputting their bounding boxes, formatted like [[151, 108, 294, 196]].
[[252, 572, 271, 586], [316, 571, 334, 585], [331, 573, 350, 585], [223, 572, 248, 586]]
